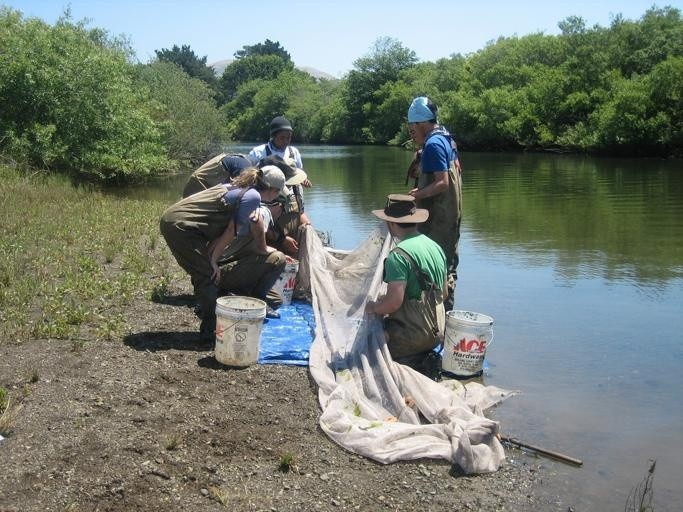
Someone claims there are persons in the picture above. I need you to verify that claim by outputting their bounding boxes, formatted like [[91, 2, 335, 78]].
[[364, 194, 450, 376], [408, 96, 462, 312], [160, 116, 313, 351]]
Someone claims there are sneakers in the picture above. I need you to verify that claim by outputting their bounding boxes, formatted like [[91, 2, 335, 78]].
[[423, 352, 443, 382]]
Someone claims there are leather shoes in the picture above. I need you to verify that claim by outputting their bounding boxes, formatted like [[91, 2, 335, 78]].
[[266, 305, 280, 318]]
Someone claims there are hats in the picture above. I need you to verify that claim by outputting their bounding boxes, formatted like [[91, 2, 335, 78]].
[[256, 155, 307, 203], [372, 193, 429, 223], [269, 117, 293, 136]]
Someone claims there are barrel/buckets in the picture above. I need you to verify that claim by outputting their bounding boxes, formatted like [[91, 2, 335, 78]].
[[268, 259, 300, 306], [214, 296, 266, 367], [441, 310, 495, 377]]
